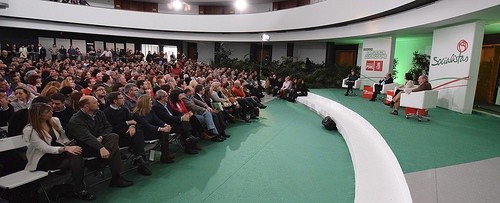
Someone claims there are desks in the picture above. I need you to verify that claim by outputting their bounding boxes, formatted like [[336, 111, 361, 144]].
[[405, 107, 427, 116], [363, 85, 373, 99], [386, 90, 394, 105]]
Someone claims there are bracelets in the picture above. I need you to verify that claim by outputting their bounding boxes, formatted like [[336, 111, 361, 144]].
[[64, 145, 66, 151]]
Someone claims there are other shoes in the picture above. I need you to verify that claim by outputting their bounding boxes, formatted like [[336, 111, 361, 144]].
[[73, 189, 97, 200], [200, 132, 216, 140], [160, 154, 175, 163], [390, 112, 398, 115], [384, 102, 393, 108], [211, 135, 226, 142], [260, 105, 267, 109], [222, 133, 230, 137], [185, 135, 201, 154]]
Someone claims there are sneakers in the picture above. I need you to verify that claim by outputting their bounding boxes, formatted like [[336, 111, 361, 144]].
[[133, 157, 146, 166], [139, 166, 152, 175], [110, 179, 133, 186]]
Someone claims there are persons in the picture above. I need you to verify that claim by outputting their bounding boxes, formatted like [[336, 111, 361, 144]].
[[182, 86, 224, 143], [23, 103, 95, 200], [0, 43, 171, 137], [67, 96, 134, 188], [168, 89, 213, 151], [153, 90, 200, 155], [168, 51, 268, 129], [393, 72, 414, 99], [133, 94, 176, 164], [194, 84, 231, 140], [265, 72, 310, 102], [103, 91, 153, 176], [345, 69, 358, 96], [383, 74, 432, 115], [369, 73, 394, 101]]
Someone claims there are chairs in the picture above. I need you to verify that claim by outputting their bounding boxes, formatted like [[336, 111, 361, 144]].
[[400, 90, 438, 122], [373, 82, 396, 102], [395, 85, 420, 108], [0, 135, 51, 203], [342, 76, 361, 96]]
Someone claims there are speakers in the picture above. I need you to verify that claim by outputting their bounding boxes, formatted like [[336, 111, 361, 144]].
[[322, 116, 337, 130]]
